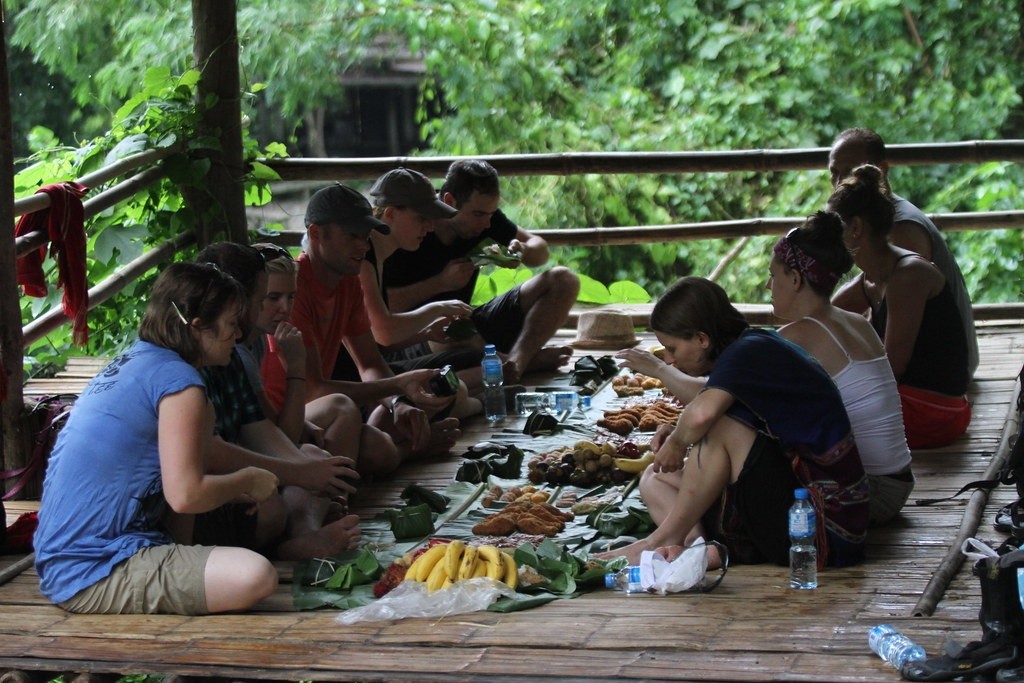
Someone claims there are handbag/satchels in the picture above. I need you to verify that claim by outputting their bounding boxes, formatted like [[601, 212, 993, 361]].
[[34, 396, 69, 464]]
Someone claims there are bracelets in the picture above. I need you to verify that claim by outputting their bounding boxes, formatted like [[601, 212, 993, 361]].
[[286, 374, 308, 384], [386, 393, 413, 416]]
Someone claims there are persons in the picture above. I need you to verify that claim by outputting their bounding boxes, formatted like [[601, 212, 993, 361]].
[[243, 242, 363, 525], [258, 184, 463, 477], [329, 168, 529, 428], [187, 244, 363, 563], [826, 127, 976, 395], [614, 209, 918, 538], [821, 164, 979, 449], [32, 261, 282, 617], [385, 158, 581, 393], [591, 276, 871, 572]]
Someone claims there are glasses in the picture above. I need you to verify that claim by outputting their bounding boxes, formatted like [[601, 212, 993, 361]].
[[786, 226, 803, 273], [194, 262, 221, 318], [260, 247, 295, 266], [682, 540, 730, 593]]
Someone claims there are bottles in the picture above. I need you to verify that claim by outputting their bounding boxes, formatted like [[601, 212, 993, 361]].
[[866, 624, 928, 673], [515, 391, 590, 415], [605, 566, 640, 592], [481, 344, 506, 421], [789, 489, 817, 591]]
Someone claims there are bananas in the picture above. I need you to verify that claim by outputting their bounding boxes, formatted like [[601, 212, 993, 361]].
[[404, 540, 519, 595], [574, 440, 600, 455], [615, 450, 655, 472]]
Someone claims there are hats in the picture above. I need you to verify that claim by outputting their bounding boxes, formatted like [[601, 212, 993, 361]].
[[304, 183, 391, 236], [568, 309, 644, 349], [368, 167, 459, 219]]
